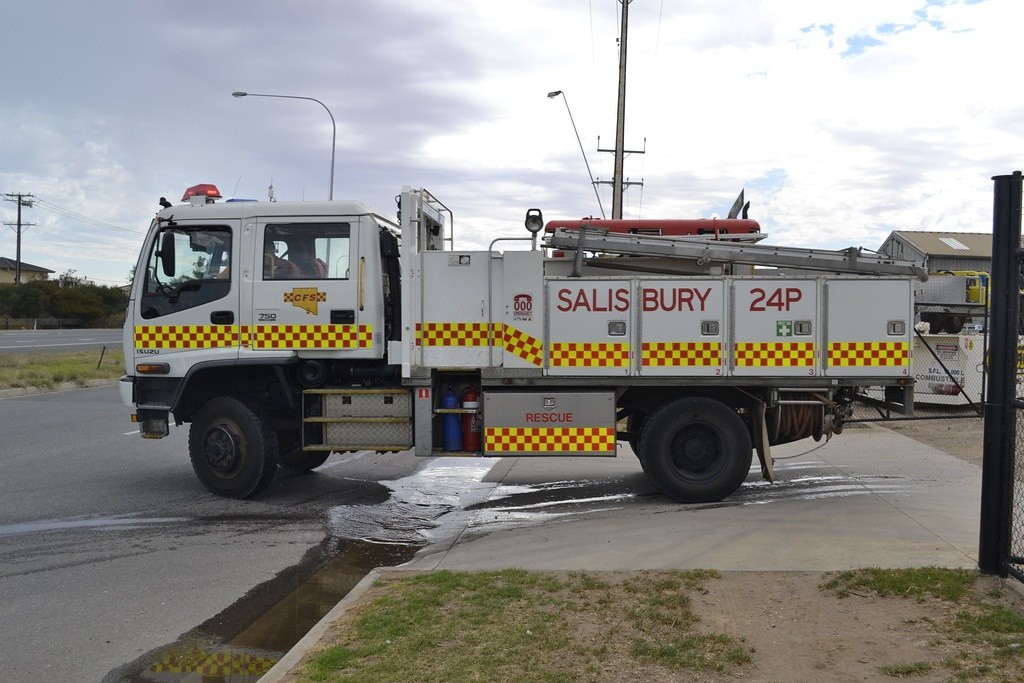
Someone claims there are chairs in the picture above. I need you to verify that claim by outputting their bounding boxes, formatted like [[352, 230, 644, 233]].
[[264, 258, 326, 278]]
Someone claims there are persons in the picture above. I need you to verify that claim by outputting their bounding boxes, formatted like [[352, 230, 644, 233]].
[[263, 237, 289, 274]]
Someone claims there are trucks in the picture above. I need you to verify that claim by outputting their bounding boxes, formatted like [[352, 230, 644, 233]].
[[119, 183, 934, 504]]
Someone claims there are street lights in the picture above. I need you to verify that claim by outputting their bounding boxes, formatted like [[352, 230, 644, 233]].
[[547, 90, 607, 219], [231, 90, 337, 281]]
